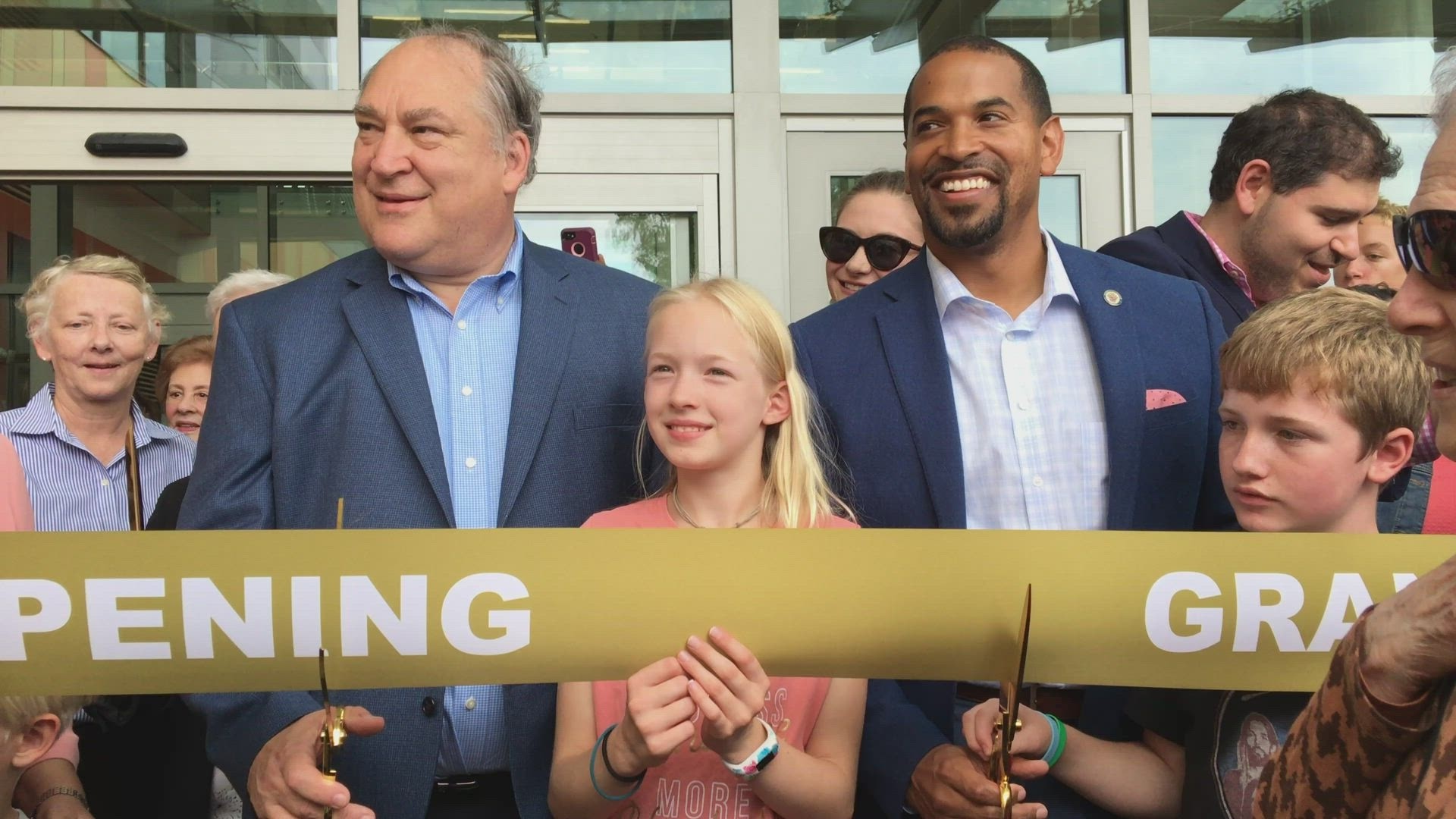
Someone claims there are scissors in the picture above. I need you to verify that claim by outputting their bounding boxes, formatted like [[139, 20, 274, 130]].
[[318, 647, 346, 819], [124, 426, 144, 531], [984, 582, 1032, 818]]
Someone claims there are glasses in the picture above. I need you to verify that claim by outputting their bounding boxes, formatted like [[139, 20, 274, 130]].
[[1391, 209, 1456, 291], [819, 226, 925, 273]]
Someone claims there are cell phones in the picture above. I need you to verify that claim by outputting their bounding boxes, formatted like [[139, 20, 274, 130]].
[[560, 227, 598, 262]]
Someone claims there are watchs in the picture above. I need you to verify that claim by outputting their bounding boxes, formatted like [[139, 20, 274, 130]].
[[722, 717, 778, 780]]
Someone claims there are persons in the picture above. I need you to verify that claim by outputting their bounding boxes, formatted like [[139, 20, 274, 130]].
[[0, 23, 1456, 819]]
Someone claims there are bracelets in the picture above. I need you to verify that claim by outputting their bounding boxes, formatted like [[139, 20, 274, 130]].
[[590, 724, 645, 801], [1034, 710, 1060, 763], [30, 786, 90, 819], [1044, 713, 1067, 767], [601, 730, 645, 782]]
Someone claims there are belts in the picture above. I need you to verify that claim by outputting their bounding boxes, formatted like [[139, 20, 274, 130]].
[[427, 773, 516, 818]]
